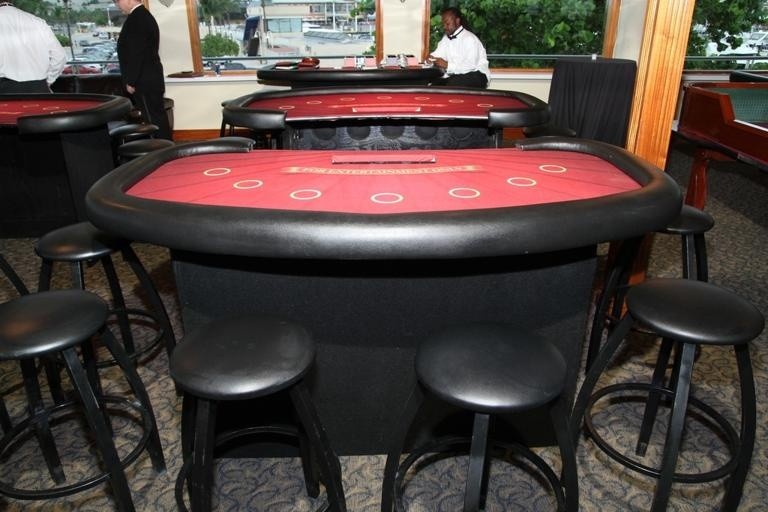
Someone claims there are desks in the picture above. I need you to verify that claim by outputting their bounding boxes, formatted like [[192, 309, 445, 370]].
[[681, 78, 768, 215], [0, 91, 132, 239], [84, 58, 686, 455]]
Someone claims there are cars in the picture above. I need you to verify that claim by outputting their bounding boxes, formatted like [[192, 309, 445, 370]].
[[63, 38, 121, 73], [92, 31, 109, 38]]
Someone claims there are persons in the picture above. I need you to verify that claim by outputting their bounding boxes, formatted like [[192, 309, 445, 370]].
[[426, 6, 491, 90], [0, 1, 69, 94], [111, 0, 173, 143]]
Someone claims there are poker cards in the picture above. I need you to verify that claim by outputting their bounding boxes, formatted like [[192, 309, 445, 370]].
[[331, 154, 436, 163], [354, 107, 421, 112]]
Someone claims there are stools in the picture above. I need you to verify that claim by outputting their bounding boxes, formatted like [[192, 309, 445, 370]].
[[570, 205, 768, 512], [381, 319, 580, 511], [166, 315, 347, 512], [1, 220, 177, 512]]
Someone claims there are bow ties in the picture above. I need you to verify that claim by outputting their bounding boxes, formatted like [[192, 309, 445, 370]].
[[447, 35, 456, 41]]
